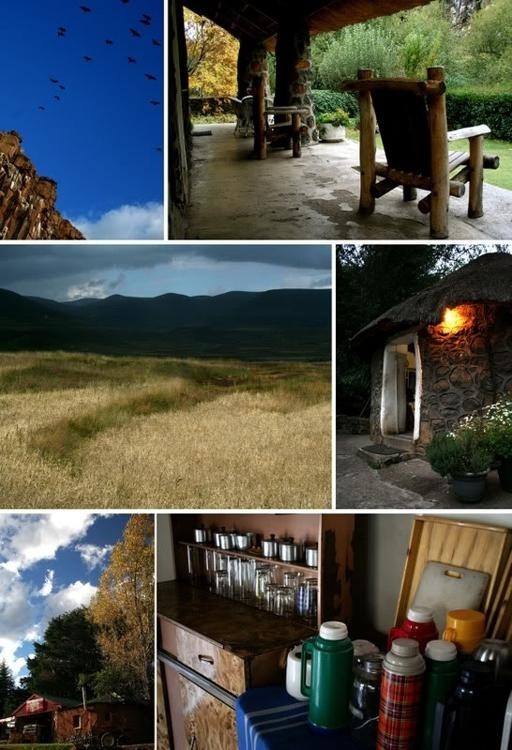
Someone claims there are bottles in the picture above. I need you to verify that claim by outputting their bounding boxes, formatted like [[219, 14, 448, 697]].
[[217, 553, 256, 600], [283, 571, 300, 589], [246, 529, 256, 548]]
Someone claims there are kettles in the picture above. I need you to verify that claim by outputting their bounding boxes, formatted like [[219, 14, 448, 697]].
[[349, 653, 384, 722]]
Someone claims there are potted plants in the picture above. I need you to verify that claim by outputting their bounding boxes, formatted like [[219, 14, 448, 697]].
[[316, 109, 351, 142]]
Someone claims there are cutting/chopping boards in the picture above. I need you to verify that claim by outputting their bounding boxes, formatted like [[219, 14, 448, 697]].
[[412, 560, 492, 638]]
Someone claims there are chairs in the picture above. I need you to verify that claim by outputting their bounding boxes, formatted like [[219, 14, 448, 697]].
[[251, 70, 308, 159], [353, 66, 501, 240], [227, 95, 274, 138]]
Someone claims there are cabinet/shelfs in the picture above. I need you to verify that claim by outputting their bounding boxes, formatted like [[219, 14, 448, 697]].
[[168, 514, 368, 632]]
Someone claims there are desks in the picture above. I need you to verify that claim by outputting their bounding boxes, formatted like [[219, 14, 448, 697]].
[[236, 655, 379, 750], [157, 579, 316, 749]]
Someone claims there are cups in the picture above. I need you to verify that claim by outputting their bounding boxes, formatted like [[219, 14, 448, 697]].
[[264, 583, 279, 611], [254, 569, 269, 599], [214, 570, 231, 596], [305, 576, 318, 620], [273, 586, 294, 616]]
